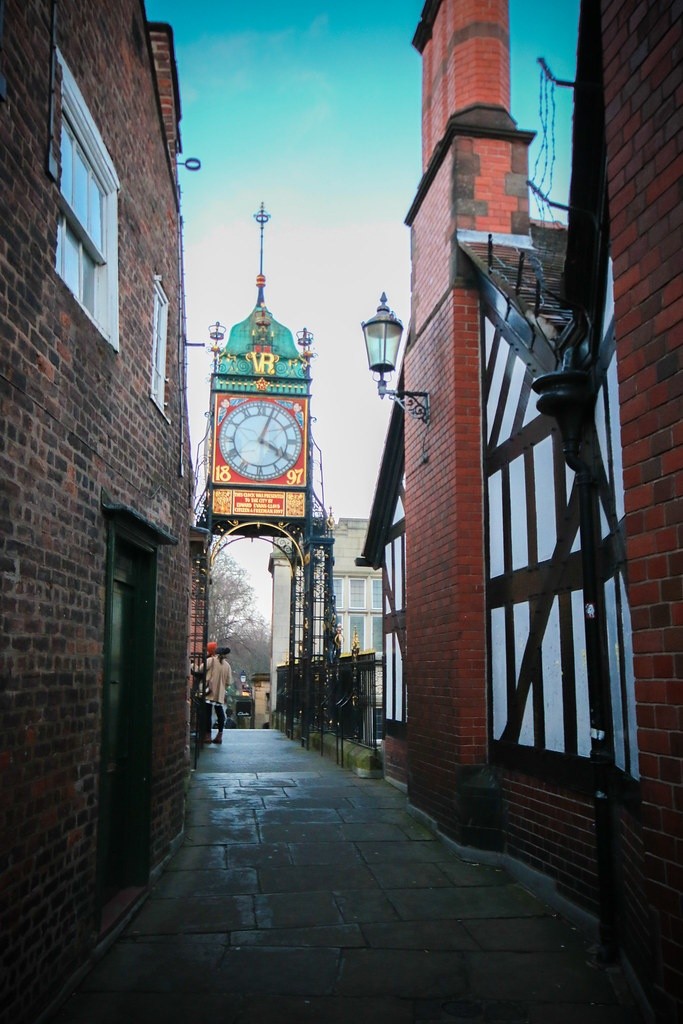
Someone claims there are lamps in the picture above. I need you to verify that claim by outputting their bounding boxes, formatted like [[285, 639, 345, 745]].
[[360, 292, 430, 462]]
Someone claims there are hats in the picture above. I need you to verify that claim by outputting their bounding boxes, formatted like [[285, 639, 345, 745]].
[[216, 647, 230, 655]]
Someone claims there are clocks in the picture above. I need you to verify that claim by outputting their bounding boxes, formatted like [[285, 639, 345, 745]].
[[218, 400, 303, 481]]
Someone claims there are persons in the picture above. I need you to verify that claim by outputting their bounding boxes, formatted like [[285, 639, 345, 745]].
[[191, 646, 234, 744]]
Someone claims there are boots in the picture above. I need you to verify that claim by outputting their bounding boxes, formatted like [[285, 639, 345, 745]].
[[211, 732, 222, 743], [204, 733, 211, 742]]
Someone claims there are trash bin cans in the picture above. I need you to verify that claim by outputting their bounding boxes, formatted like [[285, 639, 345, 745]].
[[263, 722, 269, 729]]
